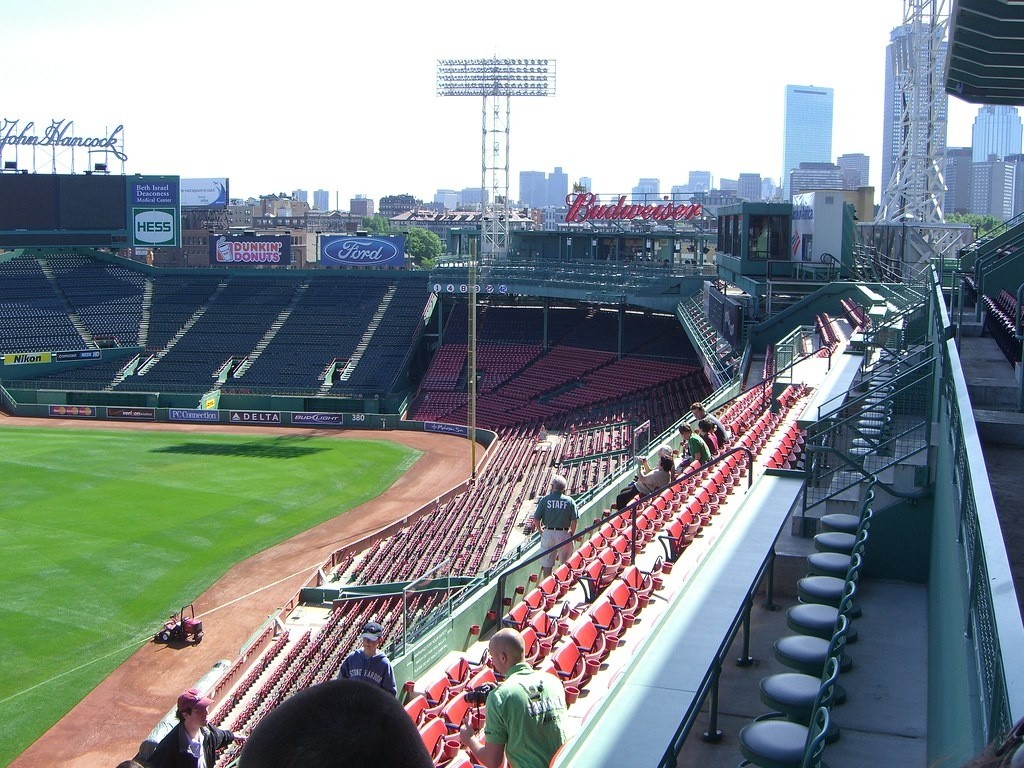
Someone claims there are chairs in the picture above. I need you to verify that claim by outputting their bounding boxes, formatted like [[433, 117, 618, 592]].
[[208, 244, 1024, 768]]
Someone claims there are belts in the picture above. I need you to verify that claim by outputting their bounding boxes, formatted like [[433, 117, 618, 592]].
[[543, 527, 569, 531]]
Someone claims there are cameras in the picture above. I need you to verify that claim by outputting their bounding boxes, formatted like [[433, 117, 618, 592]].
[[464, 685, 493, 705]]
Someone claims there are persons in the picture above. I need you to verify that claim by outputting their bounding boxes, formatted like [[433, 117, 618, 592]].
[[237, 678, 436, 768], [337, 622, 397, 698], [115, 688, 247, 768], [459, 628, 570, 768], [533, 475, 580, 579], [615, 402, 729, 512]]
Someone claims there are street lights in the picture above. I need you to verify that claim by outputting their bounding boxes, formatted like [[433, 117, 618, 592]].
[[434, 55, 555, 260]]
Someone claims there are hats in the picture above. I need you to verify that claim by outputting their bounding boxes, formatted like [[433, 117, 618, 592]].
[[178, 689, 217, 710], [360, 622, 383, 643]]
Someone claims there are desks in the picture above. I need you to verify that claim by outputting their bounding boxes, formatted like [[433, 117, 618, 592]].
[[868, 303, 887, 327], [548, 469, 807, 768], [796, 351, 865, 486], [792, 263, 839, 279], [854, 284, 885, 311], [410, 651, 465, 701]]
